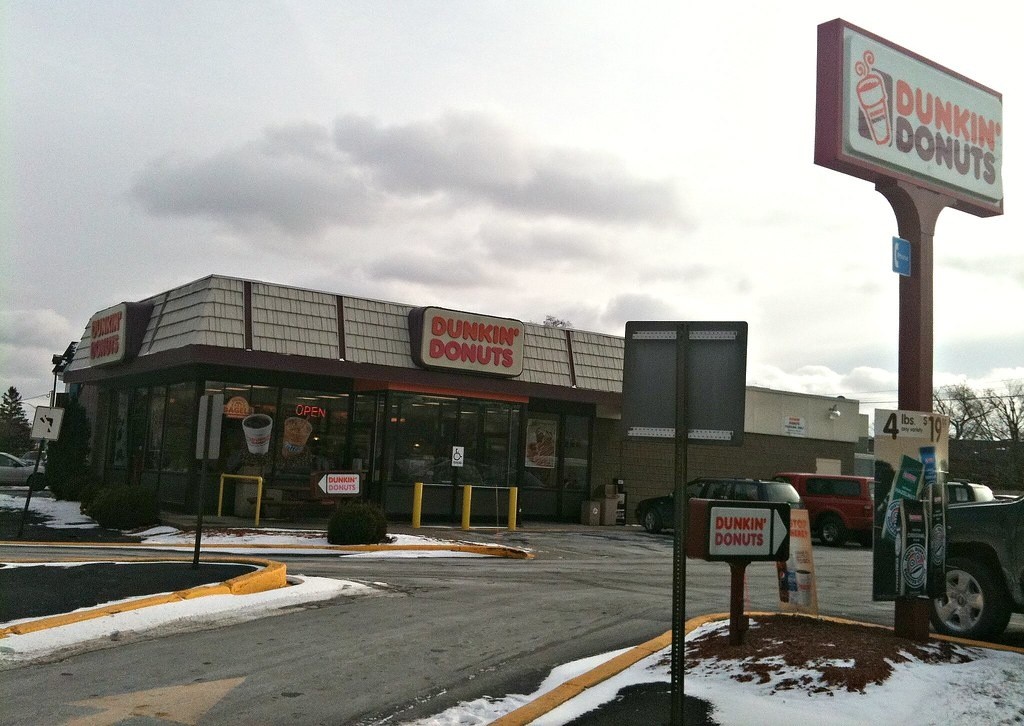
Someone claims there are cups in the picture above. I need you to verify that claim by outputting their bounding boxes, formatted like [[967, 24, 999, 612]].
[[282, 417, 313, 458], [242, 414, 273, 454]]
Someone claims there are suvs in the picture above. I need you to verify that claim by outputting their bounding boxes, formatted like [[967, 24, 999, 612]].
[[771, 472, 876, 549], [634, 475, 802, 534]]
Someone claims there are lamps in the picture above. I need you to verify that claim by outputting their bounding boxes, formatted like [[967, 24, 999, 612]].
[[829, 404, 841, 421]]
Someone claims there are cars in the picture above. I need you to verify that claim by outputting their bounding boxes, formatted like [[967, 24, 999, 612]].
[[20, 450, 48, 466], [928, 495, 1024, 642], [0, 452, 48, 491], [948, 481, 994, 504]]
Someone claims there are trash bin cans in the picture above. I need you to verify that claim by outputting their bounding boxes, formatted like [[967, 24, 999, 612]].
[[582, 479, 628, 525], [234, 465, 264, 518]]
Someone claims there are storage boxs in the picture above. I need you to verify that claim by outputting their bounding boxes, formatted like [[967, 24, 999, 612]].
[[593, 496, 619, 512], [581, 501, 600, 525], [600, 511, 618, 526], [597, 484, 618, 499]]
